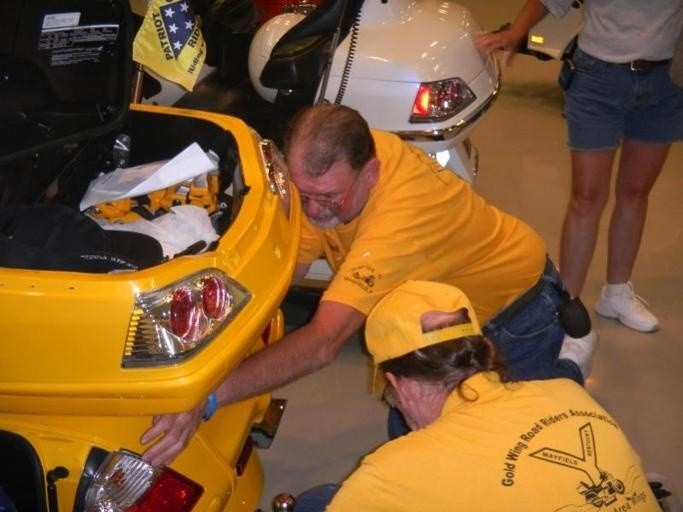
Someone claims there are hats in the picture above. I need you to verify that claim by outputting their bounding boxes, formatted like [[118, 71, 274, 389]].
[[364, 279, 483, 395]]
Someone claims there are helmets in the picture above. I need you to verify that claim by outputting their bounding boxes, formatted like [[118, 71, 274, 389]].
[[248, 12, 323, 105]]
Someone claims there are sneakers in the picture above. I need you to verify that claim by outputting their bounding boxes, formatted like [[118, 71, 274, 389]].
[[558, 330, 597, 381], [595, 285, 660, 332]]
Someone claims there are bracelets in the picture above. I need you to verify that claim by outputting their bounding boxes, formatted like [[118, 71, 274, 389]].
[[201, 392, 218, 422]]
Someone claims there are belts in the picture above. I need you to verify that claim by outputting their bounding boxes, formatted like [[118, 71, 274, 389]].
[[487, 253, 553, 330], [622, 58, 675, 68]]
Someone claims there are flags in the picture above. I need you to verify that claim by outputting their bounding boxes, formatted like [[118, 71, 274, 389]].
[[132, 1, 207, 93]]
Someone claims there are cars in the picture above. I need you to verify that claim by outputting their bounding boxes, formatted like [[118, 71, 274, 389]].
[[1, 2, 300, 512], [291, 0, 508, 305]]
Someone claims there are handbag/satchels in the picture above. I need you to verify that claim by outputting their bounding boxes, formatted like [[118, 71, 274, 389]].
[[559, 294, 593, 338]]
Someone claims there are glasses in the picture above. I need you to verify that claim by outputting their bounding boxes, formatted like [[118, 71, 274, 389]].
[[300, 177, 357, 211], [380, 383, 398, 409]]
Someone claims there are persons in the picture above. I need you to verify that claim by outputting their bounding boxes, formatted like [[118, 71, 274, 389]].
[[142, 106, 599, 467], [476, 0, 683, 333], [294, 280, 663, 512]]
